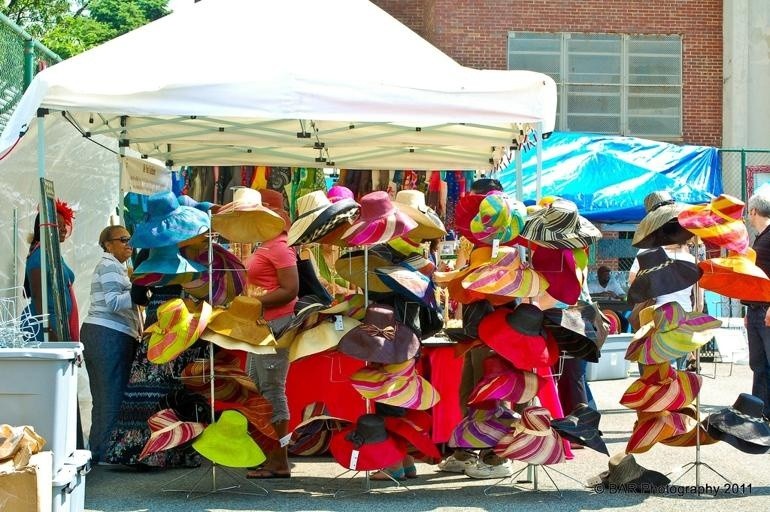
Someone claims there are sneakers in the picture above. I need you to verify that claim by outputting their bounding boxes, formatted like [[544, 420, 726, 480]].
[[438, 456, 512, 479]]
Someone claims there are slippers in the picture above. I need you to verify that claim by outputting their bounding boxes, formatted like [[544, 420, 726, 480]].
[[246, 469, 290, 479]]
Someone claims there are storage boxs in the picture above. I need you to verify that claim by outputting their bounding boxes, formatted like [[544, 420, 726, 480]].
[[584, 334, 635, 381], [0, 341, 92, 512]]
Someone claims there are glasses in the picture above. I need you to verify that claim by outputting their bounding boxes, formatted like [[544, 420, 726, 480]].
[[112, 239, 130, 242]]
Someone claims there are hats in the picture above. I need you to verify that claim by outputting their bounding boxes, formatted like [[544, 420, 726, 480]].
[[582, 191, 770, 491], [128, 185, 607, 470]]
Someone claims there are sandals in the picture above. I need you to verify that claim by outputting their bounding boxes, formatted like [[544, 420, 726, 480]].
[[369, 466, 416, 480]]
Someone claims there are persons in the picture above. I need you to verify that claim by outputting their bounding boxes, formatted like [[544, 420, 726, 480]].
[[587, 266, 628, 333], [438, 178, 517, 478], [20, 202, 83, 450], [740, 192, 770, 417], [79, 225, 146, 465], [539, 246, 597, 450], [628, 243, 704, 377], [245, 190, 299, 478], [369, 291, 423, 480]]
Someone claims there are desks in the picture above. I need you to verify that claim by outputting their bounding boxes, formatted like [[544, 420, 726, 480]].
[[594, 298, 634, 334]]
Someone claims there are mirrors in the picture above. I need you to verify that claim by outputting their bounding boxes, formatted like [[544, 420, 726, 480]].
[[41, 177, 71, 342]]
